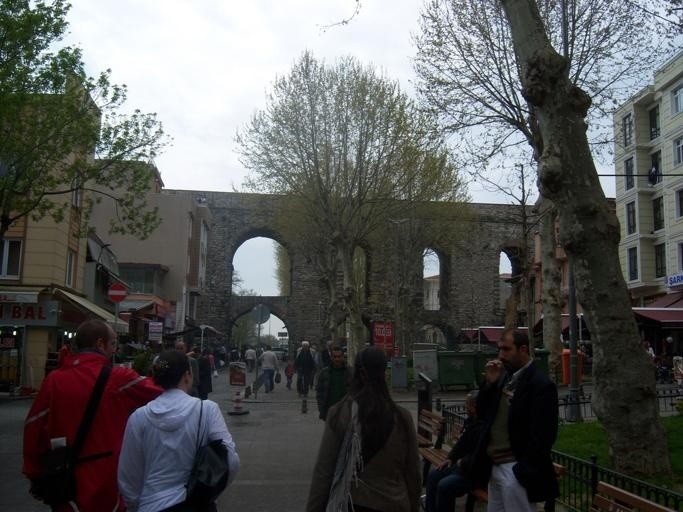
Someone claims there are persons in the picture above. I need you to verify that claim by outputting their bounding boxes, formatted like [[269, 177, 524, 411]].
[[20, 318, 164, 511], [653, 356, 666, 385], [303, 343, 423, 512], [473, 322, 560, 512], [56, 332, 346, 416], [424, 388, 493, 512], [644, 341, 655, 362], [314, 346, 355, 424], [117, 346, 239, 512]]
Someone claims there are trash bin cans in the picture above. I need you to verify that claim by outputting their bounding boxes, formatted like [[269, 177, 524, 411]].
[[412, 342, 551, 394], [559, 348, 585, 385]]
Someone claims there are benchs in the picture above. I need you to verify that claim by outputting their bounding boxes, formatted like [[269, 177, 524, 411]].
[[589, 481, 676, 512], [416, 408, 447, 448], [417, 423, 566, 512]]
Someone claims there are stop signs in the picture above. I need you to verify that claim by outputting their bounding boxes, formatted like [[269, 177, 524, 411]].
[[109, 284, 128, 302]]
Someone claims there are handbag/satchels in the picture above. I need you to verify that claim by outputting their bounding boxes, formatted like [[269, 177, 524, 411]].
[[28, 443, 81, 507], [186, 438, 232, 510], [325, 399, 365, 512], [275, 372, 283, 383]]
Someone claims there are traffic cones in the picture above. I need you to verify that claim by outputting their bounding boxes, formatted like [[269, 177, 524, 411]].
[[228, 394, 250, 415]]
